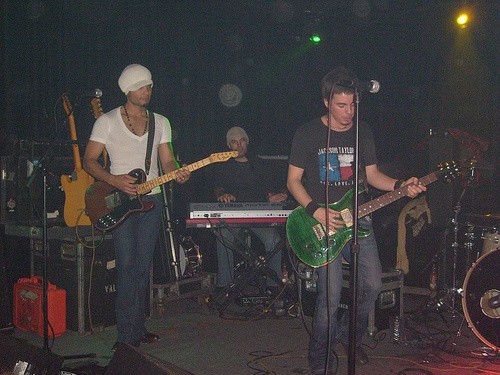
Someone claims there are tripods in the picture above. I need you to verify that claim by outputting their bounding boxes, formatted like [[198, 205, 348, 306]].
[[422, 169, 475, 322]]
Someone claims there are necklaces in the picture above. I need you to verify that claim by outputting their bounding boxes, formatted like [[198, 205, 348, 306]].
[[123, 105, 149, 136]]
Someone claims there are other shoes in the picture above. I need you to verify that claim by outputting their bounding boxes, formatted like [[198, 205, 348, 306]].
[[343, 342, 369, 366]]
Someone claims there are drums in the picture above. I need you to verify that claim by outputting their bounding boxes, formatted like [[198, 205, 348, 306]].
[[461, 247, 500, 353], [177, 235, 202, 279], [480, 227, 500, 257]]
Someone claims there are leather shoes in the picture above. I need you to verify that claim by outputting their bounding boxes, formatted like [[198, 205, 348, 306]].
[[111, 332, 161, 352]]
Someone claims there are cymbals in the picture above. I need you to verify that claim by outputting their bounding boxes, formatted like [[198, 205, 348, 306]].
[[464, 214, 500, 219], [446, 126, 496, 170]]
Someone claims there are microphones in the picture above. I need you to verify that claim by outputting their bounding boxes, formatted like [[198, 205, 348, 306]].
[[489, 294, 500, 309], [65, 89, 102, 98], [337, 80, 380, 94]]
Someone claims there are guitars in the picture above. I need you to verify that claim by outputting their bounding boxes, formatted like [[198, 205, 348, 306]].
[[285, 161, 461, 268], [87, 97, 108, 168], [60, 93, 95, 227], [84, 149, 241, 232]]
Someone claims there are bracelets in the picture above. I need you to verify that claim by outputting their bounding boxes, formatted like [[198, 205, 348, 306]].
[[394, 180, 404, 190], [305, 200, 321, 216]]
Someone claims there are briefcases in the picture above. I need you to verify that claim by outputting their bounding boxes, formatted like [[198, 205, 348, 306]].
[[13, 275, 66, 338]]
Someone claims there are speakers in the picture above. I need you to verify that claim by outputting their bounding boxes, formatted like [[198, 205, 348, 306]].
[[16, 142, 85, 227], [0, 332, 191, 375]]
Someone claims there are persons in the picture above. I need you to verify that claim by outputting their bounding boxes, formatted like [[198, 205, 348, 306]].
[[83, 63, 192, 355], [208, 126, 288, 304], [287, 66, 427, 375]]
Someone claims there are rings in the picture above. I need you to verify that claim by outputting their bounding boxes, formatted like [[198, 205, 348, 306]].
[[182, 178, 185, 180]]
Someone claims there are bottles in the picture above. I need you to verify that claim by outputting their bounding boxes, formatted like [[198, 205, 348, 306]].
[[281, 265, 289, 284]]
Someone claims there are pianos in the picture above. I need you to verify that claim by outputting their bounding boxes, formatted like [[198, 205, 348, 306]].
[[187, 201, 300, 314]]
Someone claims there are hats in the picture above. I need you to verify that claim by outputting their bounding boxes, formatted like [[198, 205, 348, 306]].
[[226, 126, 250, 150], [117, 63, 154, 95]]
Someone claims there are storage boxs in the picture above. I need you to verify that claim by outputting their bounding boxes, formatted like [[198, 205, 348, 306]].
[[298, 263, 404, 336], [30, 235, 153, 337]]
